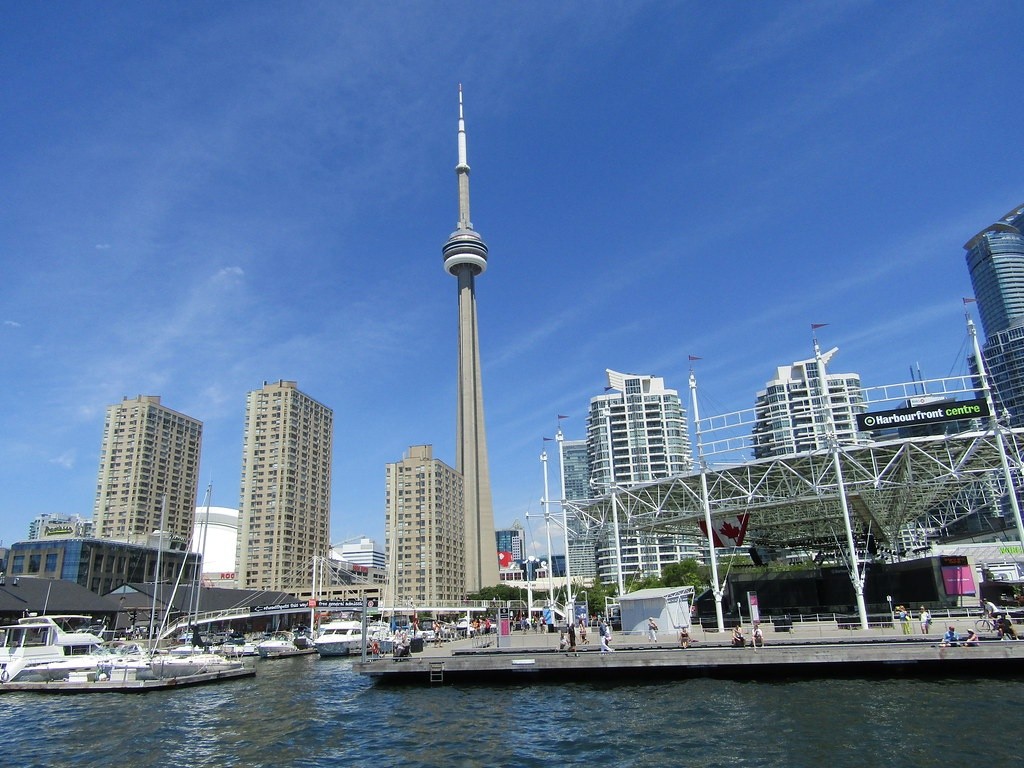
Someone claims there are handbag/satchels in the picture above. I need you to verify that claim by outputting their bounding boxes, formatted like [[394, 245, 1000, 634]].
[[682, 637, 688, 640], [905, 615, 910, 621], [928, 620, 932, 625], [533, 623, 537, 627], [606, 635, 611, 641]]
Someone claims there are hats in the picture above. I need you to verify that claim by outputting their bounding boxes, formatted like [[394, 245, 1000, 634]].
[[949, 626, 955, 630], [604, 623, 608, 626], [967, 629, 974, 634], [982, 598, 986, 602], [649, 617, 653, 620]]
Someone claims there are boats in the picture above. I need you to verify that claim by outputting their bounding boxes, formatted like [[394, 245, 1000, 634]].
[[21, 639, 152, 680], [171, 632, 260, 658], [314, 618, 376, 657], [148, 645, 243, 679], [0, 577, 107, 681], [256, 631, 317, 659]]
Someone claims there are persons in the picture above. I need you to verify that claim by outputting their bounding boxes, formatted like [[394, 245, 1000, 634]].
[[648, 617, 656, 643], [938, 626, 959, 647], [963, 628, 979, 646], [471, 617, 491, 637], [918, 607, 931, 634], [899, 606, 911, 635], [731, 625, 746, 647], [599, 619, 613, 655], [980, 598, 1000, 630], [752, 625, 764, 651], [559, 619, 586, 657], [997, 614, 1020, 640], [392, 626, 410, 660], [433, 620, 444, 648], [520, 615, 546, 634], [681, 630, 690, 649]]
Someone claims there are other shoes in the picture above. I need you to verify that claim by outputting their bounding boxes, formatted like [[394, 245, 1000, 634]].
[[1016, 638, 1019, 640], [565, 654, 569, 657], [574, 654, 580, 657], [1001, 638, 1003, 641]]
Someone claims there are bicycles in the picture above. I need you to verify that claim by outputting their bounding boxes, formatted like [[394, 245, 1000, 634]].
[[975, 615, 995, 633]]
